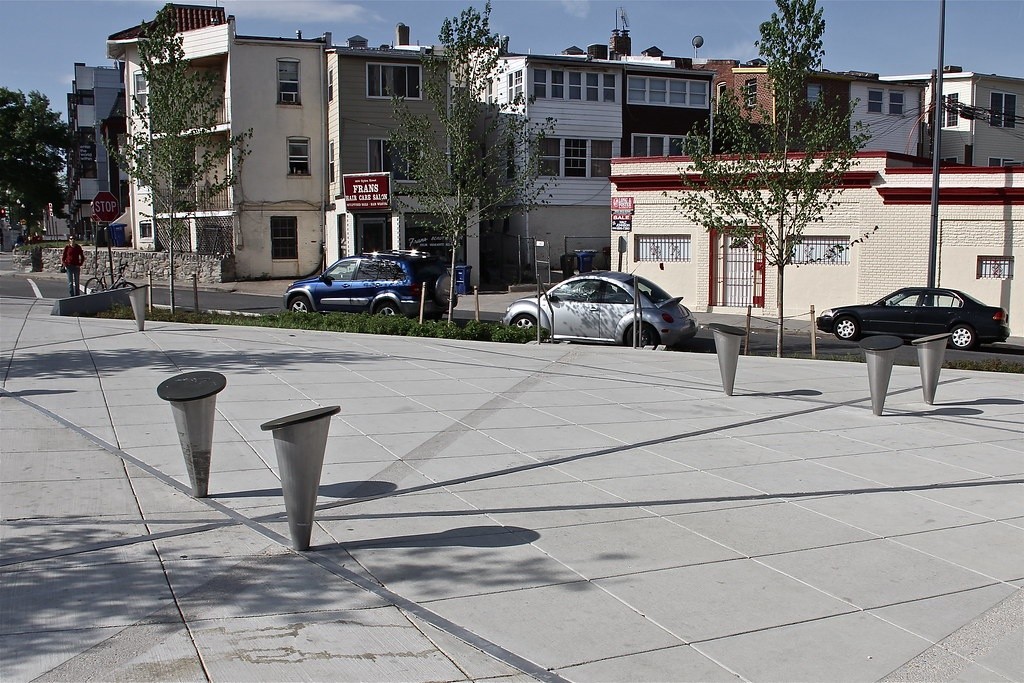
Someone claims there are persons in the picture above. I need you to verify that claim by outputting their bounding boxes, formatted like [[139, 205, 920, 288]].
[[16, 232, 43, 246], [61, 235, 85, 296]]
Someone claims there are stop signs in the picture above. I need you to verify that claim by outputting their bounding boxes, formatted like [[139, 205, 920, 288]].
[[93, 191, 119, 223]]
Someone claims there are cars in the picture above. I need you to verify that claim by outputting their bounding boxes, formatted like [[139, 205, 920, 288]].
[[816, 286, 1011, 349], [503, 270, 700, 348]]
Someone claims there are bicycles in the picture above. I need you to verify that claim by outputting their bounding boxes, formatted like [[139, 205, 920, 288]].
[[84, 261, 137, 295]]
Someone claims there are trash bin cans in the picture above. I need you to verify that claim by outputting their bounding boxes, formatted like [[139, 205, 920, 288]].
[[455, 265, 473, 295], [109, 223, 128, 247], [94, 224, 108, 247], [560, 251, 596, 280]]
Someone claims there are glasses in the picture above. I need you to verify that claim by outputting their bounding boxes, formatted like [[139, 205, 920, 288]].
[[69, 238, 75, 240]]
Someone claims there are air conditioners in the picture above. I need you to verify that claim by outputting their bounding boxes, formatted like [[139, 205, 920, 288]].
[[280, 92, 295, 102]]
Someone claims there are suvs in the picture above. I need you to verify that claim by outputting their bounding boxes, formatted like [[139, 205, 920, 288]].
[[283, 248, 458, 320]]
